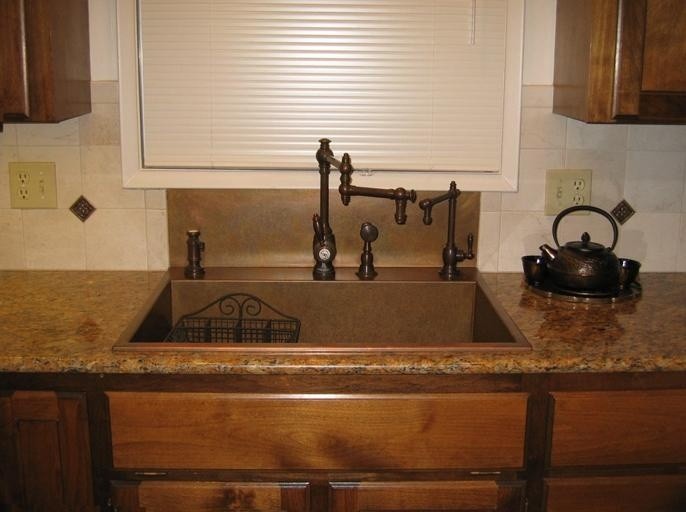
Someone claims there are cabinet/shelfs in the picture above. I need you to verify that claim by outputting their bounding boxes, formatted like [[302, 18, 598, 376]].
[[0, 0, 93, 125], [535, 373, 685, 512], [94, 373, 535, 512], [551, 0, 685, 127], [0, 390, 94, 512]]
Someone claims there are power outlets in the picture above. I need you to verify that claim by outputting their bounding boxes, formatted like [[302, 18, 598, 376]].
[[7, 161, 58, 210], [543, 167, 594, 217]]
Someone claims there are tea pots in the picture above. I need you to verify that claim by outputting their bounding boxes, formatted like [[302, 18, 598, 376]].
[[539, 205, 622, 292]]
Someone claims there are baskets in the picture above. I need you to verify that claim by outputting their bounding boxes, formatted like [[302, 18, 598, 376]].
[[162, 291, 302, 343]]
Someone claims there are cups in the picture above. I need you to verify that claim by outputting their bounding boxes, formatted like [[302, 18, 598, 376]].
[[522, 254, 542, 279], [617, 258, 642, 283]]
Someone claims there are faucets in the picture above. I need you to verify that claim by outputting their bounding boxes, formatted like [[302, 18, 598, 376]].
[[313, 135, 416, 278], [419, 179, 476, 279]]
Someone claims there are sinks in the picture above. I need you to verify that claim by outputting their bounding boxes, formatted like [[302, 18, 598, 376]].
[[111, 266, 533, 354]]
